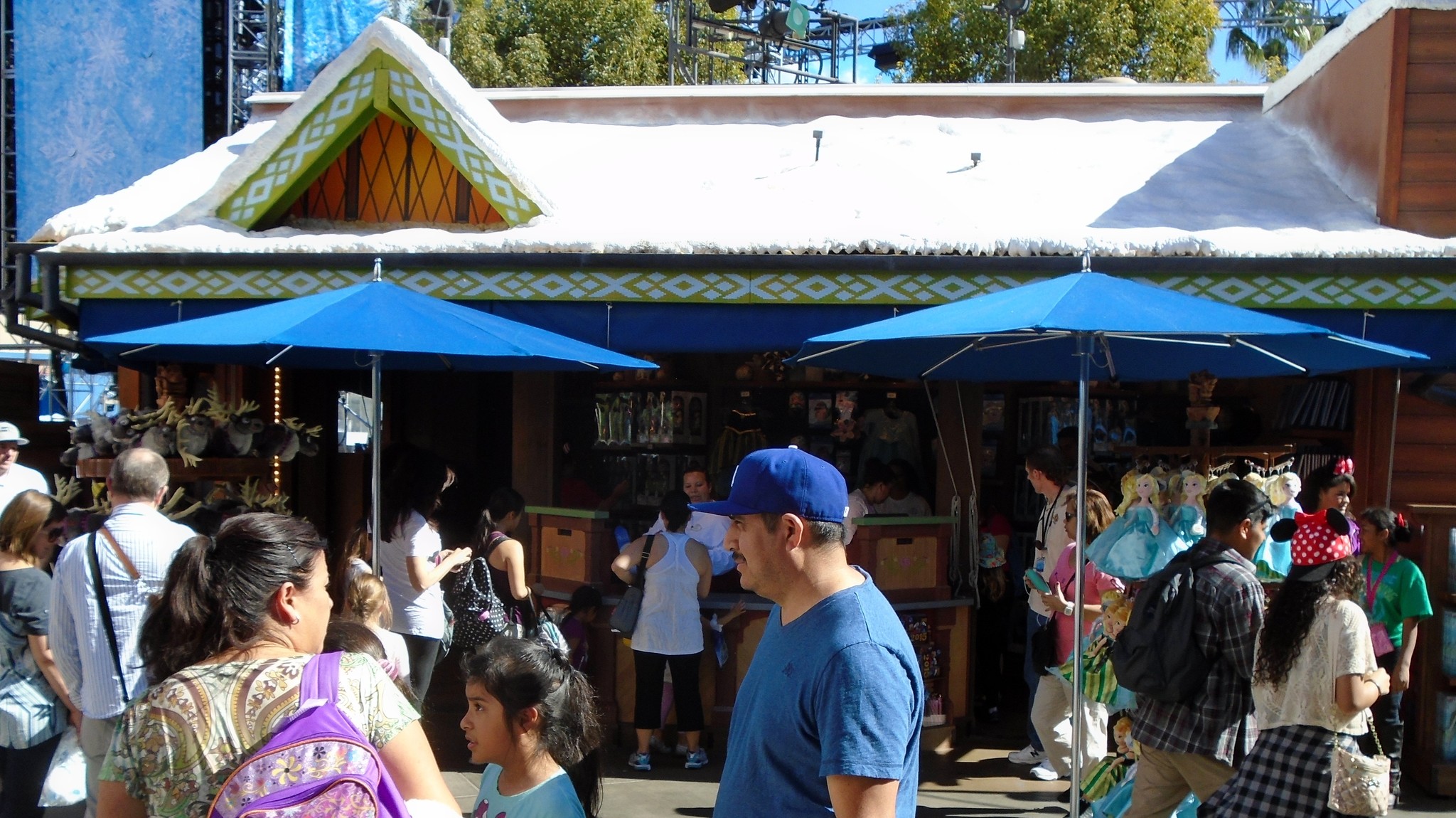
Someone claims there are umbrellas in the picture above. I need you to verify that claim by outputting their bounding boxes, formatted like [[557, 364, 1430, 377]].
[[786, 250, 1430, 818], [80, 259, 663, 576]]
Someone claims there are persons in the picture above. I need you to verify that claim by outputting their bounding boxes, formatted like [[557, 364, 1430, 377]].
[[2, 418, 609, 818], [846, 457, 898, 515], [610, 462, 734, 769], [689, 442, 923, 818], [1004, 428, 1431, 818], [877, 457, 934, 515]]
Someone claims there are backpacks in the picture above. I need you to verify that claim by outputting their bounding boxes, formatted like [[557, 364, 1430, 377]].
[[1113, 552, 1246, 701], [209, 652, 415, 818], [450, 536, 514, 647]]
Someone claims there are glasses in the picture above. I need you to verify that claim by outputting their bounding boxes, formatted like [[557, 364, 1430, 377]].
[[1065, 512, 1077, 520]]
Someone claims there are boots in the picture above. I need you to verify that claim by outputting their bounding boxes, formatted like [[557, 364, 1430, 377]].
[[1383, 721, 1404, 809]]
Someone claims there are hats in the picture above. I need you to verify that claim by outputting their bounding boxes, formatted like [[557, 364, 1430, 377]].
[[687, 445, 850, 525], [0, 422, 28, 445], [1271, 507, 1352, 581]]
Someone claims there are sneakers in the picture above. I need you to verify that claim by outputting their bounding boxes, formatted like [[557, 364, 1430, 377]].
[[1058, 785, 1083, 803], [629, 752, 651, 770], [1029, 758, 1064, 780], [685, 748, 711, 768], [1008, 745, 1047, 765], [677, 744, 688, 755], [651, 733, 670, 751]]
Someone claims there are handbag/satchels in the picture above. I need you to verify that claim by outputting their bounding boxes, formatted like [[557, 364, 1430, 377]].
[[526, 585, 569, 657], [37, 726, 89, 807], [608, 586, 643, 638], [1327, 748, 1391, 816], [1032, 621, 1055, 676]]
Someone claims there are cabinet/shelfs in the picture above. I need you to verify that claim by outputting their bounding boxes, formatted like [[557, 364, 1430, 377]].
[[588, 379, 714, 523], [1407, 500, 1456, 796], [1013, 388, 1148, 571]]
